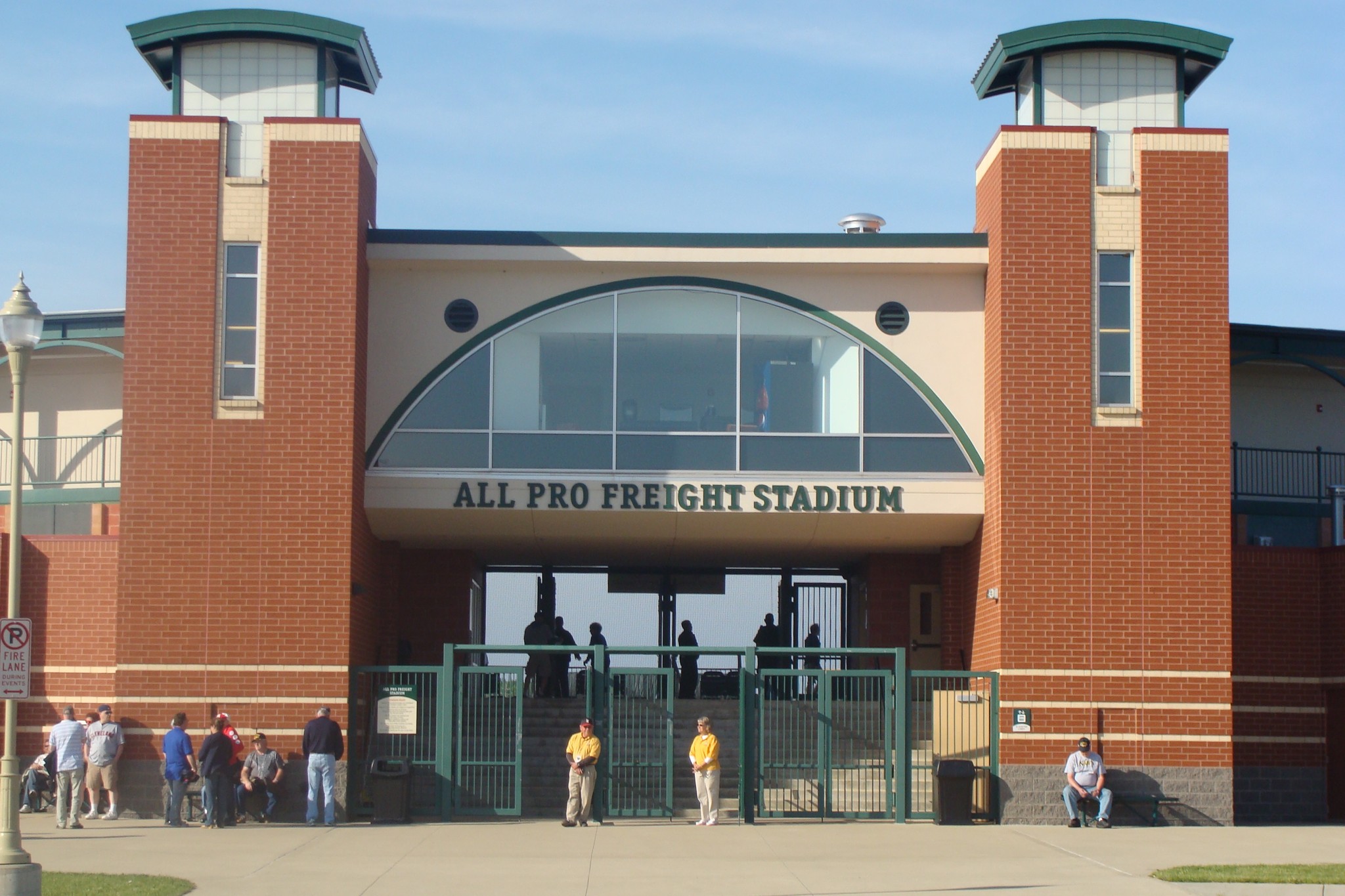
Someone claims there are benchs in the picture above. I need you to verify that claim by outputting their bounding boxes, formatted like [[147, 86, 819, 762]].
[[1061, 796, 1179, 826], [41, 786, 107, 815], [167, 792, 289, 822]]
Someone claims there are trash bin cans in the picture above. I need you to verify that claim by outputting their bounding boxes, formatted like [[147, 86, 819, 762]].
[[931, 758, 976, 826], [367, 756, 415, 825]]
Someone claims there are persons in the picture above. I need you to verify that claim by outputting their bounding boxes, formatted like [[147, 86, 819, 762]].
[[561, 718, 601, 827], [198, 717, 232, 828], [584, 623, 610, 672], [523, 612, 553, 698], [552, 617, 581, 698], [302, 707, 344, 827], [82, 705, 125, 820], [689, 716, 720, 826], [753, 613, 780, 695], [200, 758, 243, 823], [678, 620, 699, 698], [1062, 737, 1112, 829], [163, 713, 197, 827], [19, 740, 49, 813], [236, 733, 285, 823], [804, 624, 823, 700], [217, 713, 243, 826], [49, 706, 87, 829]]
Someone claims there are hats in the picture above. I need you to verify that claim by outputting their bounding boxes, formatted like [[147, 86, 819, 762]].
[[581, 718, 594, 727], [63, 706, 74, 715], [252, 733, 266, 743], [216, 712, 231, 722], [1079, 738, 1090, 751], [99, 705, 111, 712]]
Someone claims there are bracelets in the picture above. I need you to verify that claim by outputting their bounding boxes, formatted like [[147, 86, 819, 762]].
[[275, 779, 279, 782], [578, 763, 580, 766]]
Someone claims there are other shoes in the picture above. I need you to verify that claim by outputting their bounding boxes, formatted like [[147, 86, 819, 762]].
[[164, 814, 267, 830], [325, 822, 336, 826], [581, 822, 588, 827], [306, 819, 316, 826], [562, 820, 577, 826], [56, 825, 66, 829], [70, 823, 83, 828]]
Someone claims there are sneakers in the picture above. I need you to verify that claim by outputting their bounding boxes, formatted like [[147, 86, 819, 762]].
[[696, 820, 706, 826], [29, 790, 37, 798], [1096, 820, 1111, 828], [1068, 819, 1081, 827], [85, 810, 98, 819], [102, 811, 118, 820], [706, 819, 718, 826], [19, 804, 32, 813]]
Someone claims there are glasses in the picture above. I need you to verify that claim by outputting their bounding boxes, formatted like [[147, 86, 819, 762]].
[[697, 724, 704, 726], [101, 711, 112, 715], [45, 745, 49, 747], [85, 720, 92, 724]]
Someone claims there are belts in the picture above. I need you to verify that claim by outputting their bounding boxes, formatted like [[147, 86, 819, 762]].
[[309, 752, 335, 755]]
